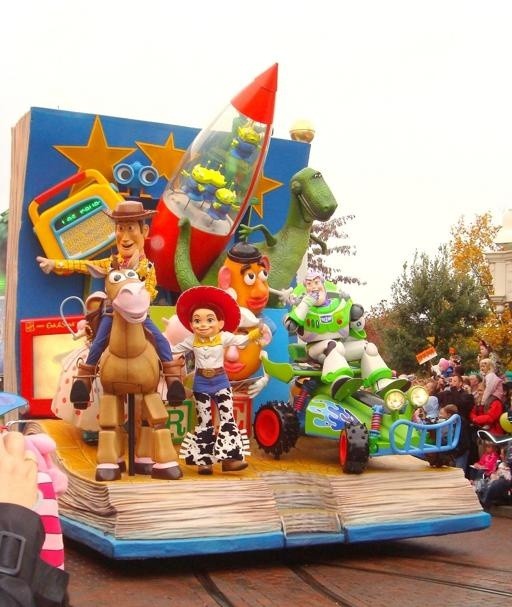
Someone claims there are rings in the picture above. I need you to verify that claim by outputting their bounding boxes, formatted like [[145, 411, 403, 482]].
[[23, 456, 40, 467]]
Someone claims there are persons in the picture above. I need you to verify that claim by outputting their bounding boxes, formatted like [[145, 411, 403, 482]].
[[392, 340, 511, 513], [0, 426, 68, 607]]
